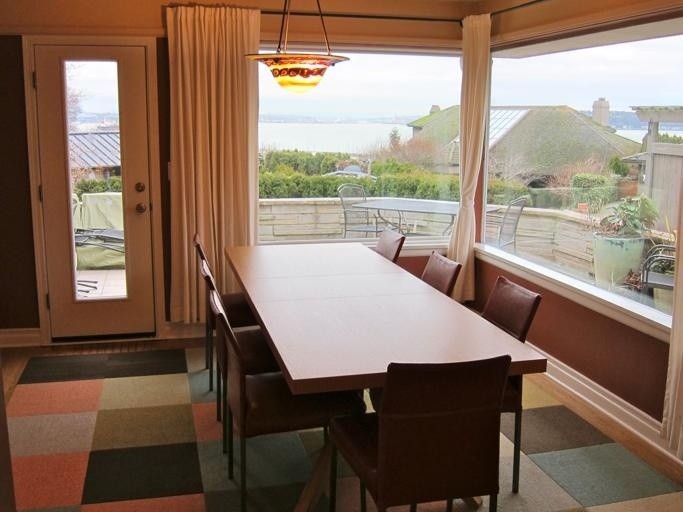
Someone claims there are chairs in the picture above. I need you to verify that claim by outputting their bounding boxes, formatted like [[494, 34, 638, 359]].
[[75, 228, 126, 293], [337, 184, 397, 238], [487, 196, 529, 254], [639, 245, 675, 307]]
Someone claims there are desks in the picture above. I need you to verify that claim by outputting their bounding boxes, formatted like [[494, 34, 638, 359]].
[[352, 198, 503, 236]]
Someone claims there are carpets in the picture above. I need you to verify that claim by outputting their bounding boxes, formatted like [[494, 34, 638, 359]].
[[6, 346, 683, 511]]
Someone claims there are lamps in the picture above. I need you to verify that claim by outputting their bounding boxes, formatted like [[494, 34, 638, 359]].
[[243, 0, 350, 94]]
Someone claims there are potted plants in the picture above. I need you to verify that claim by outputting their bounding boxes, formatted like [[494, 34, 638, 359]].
[[571, 173, 607, 212], [593, 194, 659, 287]]
